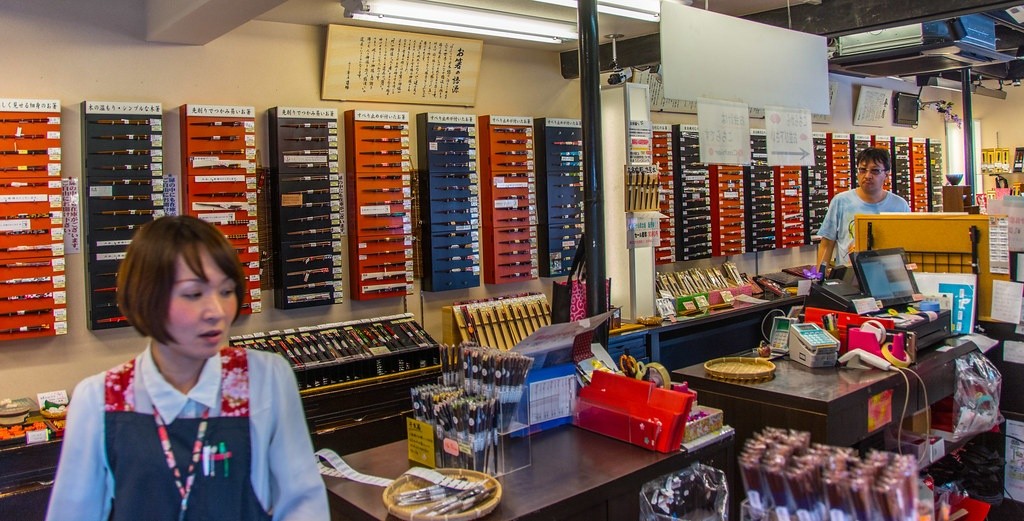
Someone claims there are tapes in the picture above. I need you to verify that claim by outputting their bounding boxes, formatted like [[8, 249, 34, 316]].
[[646, 362, 671, 389], [881, 341, 912, 368], [860, 320, 887, 344]]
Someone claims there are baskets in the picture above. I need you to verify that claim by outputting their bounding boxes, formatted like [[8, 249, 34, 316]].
[[383, 468, 502, 521], [703, 357, 776, 380]]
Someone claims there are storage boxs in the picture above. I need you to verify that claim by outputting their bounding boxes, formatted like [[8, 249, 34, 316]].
[[1002, 418, 1024, 505]]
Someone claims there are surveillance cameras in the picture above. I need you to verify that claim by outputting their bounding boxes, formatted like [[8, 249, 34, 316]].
[[607, 67, 633, 85]]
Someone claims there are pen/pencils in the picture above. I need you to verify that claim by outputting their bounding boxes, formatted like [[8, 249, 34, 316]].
[[821, 313, 839, 330]]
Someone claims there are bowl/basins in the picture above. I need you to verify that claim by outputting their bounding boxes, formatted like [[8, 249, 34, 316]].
[[946, 174, 964, 185]]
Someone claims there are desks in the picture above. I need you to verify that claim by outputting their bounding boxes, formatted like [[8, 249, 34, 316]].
[[667, 329, 998, 452], [317, 401, 737, 521]]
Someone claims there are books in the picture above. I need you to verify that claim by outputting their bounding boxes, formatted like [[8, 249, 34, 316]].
[[937, 283, 973, 335]]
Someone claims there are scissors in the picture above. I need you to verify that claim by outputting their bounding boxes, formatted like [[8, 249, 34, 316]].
[[619, 355, 646, 381]]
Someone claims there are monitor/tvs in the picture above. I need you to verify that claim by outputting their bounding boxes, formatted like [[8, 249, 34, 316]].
[[849, 247, 923, 308], [894, 92, 920, 125]]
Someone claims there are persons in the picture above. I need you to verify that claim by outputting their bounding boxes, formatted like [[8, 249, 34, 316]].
[[816, 147, 911, 281], [46, 215, 332, 521]]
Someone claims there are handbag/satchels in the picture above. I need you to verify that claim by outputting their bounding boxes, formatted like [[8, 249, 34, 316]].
[[550, 236, 613, 365]]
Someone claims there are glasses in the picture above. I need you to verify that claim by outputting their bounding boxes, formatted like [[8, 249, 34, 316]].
[[857, 167, 887, 175]]
